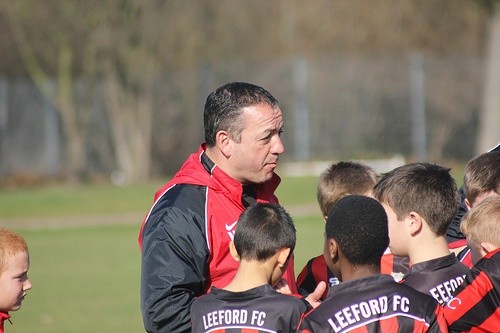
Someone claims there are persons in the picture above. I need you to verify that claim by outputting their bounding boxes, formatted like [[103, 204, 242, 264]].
[[296, 195, 444, 333], [138, 82, 326, 333], [0, 228, 32, 333], [297, 160, 411, 303], [190, 203, 314, 333], [442, 146, 500, 333], [371, 162, 471, 305]]
